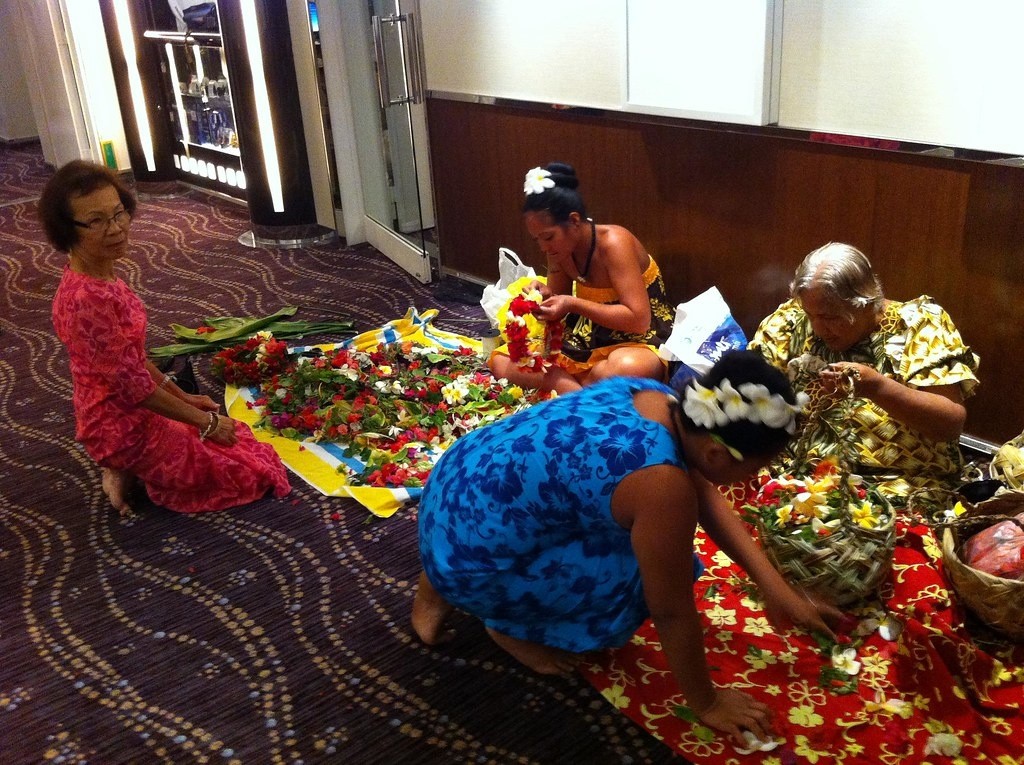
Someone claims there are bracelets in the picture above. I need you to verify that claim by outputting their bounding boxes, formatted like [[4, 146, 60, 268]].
[[198, 411, 222, 443], [159, 375, 171, 388]]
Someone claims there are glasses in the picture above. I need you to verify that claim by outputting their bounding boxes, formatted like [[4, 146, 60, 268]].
[[70, 209, 132, 230]]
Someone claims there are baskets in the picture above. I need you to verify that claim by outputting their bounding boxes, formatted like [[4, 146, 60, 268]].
[[758, 474, 896, 605], [943, 489, 1024, 640]]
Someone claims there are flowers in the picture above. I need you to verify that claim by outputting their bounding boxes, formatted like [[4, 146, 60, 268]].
[[147, 288, 964, 757], [522, 168, 555, 196]]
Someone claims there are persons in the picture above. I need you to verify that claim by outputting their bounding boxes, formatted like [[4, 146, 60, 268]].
[[747, 240, 985, 514], [490, 162, 683, 396], [412, 344, 852, 751], [39, 160, 291, 518]]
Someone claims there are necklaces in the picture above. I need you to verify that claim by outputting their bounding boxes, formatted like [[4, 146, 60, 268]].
[[570, 217, 597, 283]]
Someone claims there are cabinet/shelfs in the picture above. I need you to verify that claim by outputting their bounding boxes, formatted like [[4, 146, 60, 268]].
[[147, 0, 249, 207]]
